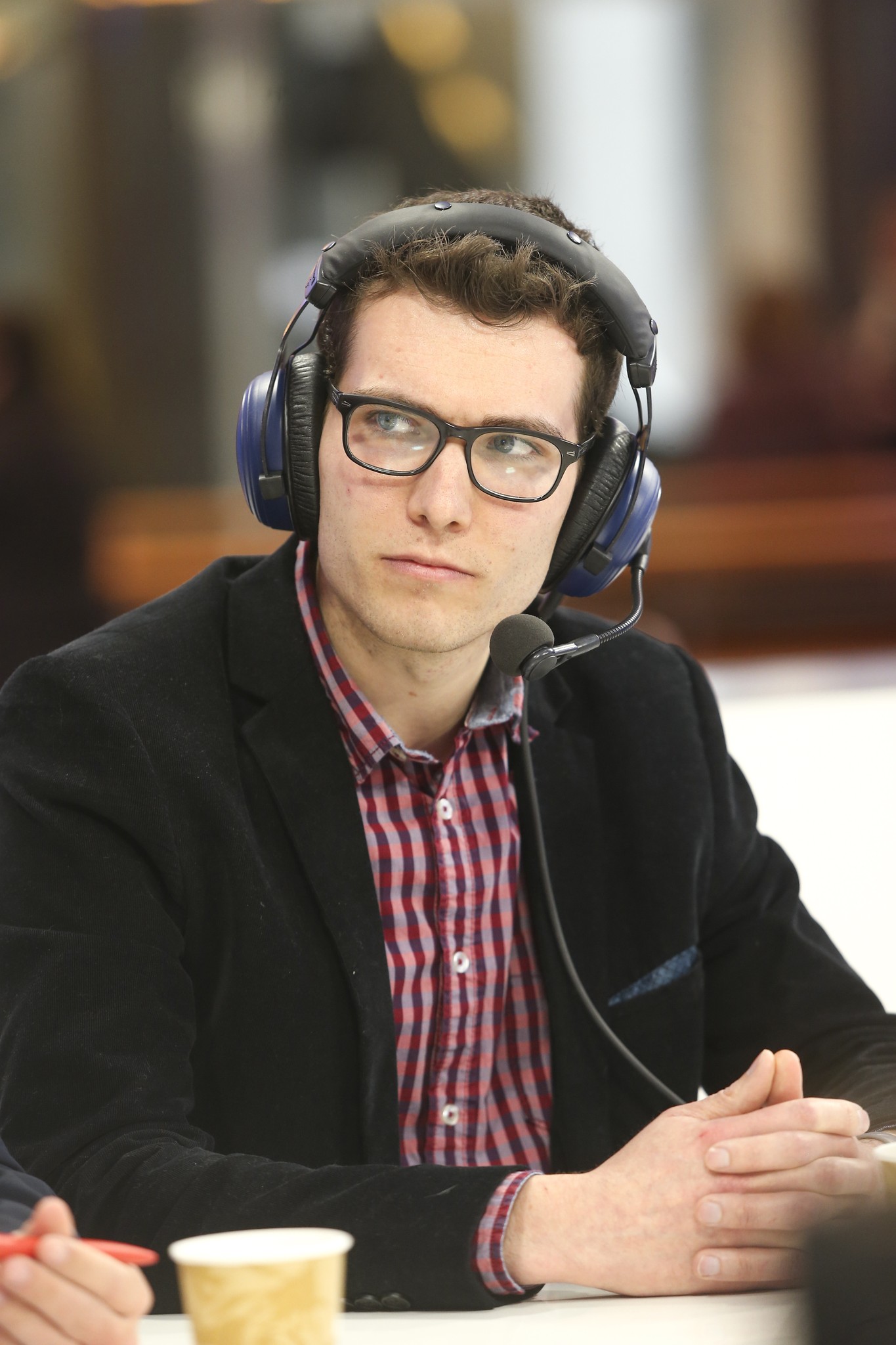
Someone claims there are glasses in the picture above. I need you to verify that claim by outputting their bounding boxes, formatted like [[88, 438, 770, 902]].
[[324, 376, 598, 504]]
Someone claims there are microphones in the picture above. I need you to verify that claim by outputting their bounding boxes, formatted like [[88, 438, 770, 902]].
[[486, 533, 650, 679]]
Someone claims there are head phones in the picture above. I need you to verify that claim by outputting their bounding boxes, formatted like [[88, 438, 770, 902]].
[[236, 199, 665, 598]]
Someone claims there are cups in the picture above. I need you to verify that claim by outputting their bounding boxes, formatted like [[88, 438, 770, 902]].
[[167, 1227, 357, 1345], [873, 1141, 896, 1227]]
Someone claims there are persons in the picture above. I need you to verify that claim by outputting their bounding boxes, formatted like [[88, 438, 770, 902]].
[[0, 1132, 154, 1345], [0, 294, 102, 687], [0, 187, 896, 1295]]
